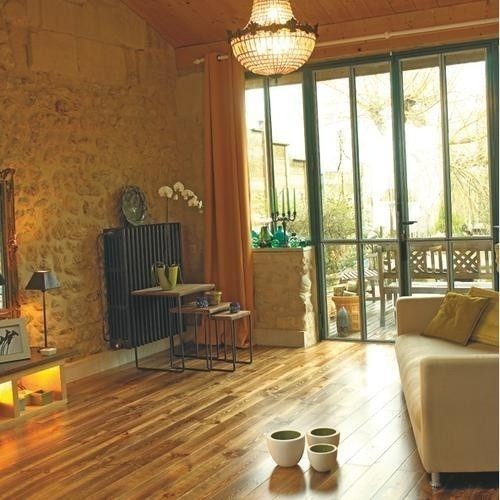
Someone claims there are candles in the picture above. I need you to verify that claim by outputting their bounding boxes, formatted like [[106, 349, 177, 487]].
[[270, 188, 298, 213]]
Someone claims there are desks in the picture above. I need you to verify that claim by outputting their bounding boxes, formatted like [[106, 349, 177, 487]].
[[129, 283, 216, 373], [168, 298, 235, 375]]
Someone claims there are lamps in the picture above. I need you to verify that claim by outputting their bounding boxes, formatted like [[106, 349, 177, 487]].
[[225, 1, 319, 86], [0, 273, 6, 287], [23, 270, 63, 358]]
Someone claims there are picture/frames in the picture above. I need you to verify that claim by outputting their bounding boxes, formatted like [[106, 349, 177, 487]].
[[0, 318, 31, 365]]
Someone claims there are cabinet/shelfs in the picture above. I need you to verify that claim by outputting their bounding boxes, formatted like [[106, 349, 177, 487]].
[[0, 347, 81, 434]]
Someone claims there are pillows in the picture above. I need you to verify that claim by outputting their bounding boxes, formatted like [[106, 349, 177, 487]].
[[464, 286, 499, 348], [421, 289, 490, 350]]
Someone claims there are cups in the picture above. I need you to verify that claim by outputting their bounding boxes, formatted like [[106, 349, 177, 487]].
[[308, 427, 340, 449], [205, 291, 222, 305]]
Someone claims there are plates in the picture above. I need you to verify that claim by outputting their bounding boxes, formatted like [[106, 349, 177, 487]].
[[121, 185, 150, 226]]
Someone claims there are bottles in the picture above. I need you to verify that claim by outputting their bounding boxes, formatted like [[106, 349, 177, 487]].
[[336, 305, 350, 337], [288, 233, 300, 248], [252, 225, 288, 249]]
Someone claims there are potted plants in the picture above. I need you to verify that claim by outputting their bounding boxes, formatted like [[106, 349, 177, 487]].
[[204, 287, 223, 305], [230, 301, 240, 313]]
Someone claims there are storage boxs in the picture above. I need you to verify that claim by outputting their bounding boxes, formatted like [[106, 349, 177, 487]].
[[29, 389, 54, 406]]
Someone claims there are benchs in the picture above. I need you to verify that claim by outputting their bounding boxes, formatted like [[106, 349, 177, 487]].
[[376, 234, 494, 329]]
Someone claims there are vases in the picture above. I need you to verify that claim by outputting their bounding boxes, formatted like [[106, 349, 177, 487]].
[[250, 225, 287, 250], [305, 426, 340, 448], [151, 262, 186, 291]]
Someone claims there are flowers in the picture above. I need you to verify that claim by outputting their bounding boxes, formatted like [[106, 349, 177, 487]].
[[156, 180, 205, 288]]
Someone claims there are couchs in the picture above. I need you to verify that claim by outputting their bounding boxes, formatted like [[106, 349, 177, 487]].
[[395, 290, 496, 490]]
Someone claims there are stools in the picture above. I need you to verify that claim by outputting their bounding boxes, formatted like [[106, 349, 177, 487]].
[[340, 268, 379, 303], [208, 308, 253, 373]]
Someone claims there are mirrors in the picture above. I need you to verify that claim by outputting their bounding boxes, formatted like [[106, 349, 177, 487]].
[[0, 167, 22, 322]]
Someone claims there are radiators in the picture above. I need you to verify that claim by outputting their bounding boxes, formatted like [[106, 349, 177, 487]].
[[101, 221, 189, 350]]
